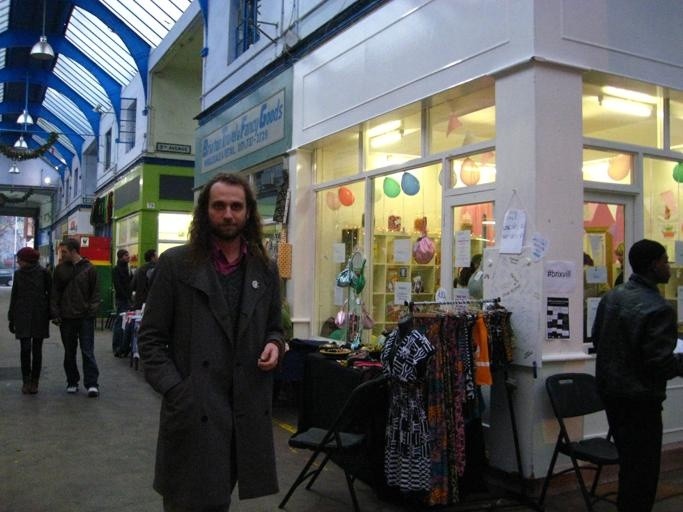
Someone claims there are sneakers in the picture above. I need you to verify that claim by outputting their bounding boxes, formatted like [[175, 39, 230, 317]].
[[134, 353, 140, 370], [119, 351, 131, 359], [88, 388, 99, 397], [114, 352, 121, 357], [66, 384, 78, 394]]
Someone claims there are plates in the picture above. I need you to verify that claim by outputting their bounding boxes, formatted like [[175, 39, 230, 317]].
[[319, 348, 350, 355]]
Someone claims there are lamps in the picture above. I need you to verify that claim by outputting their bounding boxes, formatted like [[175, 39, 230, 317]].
[[92, 104, 114, 116], [28, 1, 55, 60], [15, 72, 33, 124], [13, 126, 27, 148], [8, 153, 19, 174]]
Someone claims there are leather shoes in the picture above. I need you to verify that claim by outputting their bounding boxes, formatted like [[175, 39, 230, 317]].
[[31, 383, 37, 394], [22, 382, 31, 394]]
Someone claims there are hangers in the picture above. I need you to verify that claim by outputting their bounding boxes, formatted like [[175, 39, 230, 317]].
[[416, 298, 503, 320]]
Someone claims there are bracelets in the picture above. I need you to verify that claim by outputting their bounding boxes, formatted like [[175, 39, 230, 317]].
[[270, 340, 281, 351]]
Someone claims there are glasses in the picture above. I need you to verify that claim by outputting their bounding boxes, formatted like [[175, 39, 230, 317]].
[[117, 250, 128, 259]]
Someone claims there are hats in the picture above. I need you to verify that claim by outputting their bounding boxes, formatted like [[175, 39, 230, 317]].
[[17, 247, 38, 262], [125, 254, 128, 256], [627, 240, 665, 273]]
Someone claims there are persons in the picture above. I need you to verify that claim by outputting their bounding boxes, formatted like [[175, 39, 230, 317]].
[[52, 239, 100, 396], [584, 254, 610, 343], [591, 240, 683, 511], [137, 173, 285, 512], [135, 249, 159, 309], [112, 249, 134, 358], [8, 247, 53, 395], [613, 243, 624, 288]]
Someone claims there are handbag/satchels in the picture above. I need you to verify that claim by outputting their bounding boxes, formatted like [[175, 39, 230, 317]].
[[336, 255, 367, 293], [333, 297, 374, 343]]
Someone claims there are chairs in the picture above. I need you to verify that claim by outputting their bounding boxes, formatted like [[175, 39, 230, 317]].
[[277, 374, 387, 510], [537, 373, 621, 512]]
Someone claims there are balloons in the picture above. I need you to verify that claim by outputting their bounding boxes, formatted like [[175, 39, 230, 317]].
[[401, 170, 420, 196], [325, 189, 340, 210], [438, 164, 458, 187], [338, 186, 355, 206], [460, 158, 479, 185], [607, 154, 630, 181], [672, 161, 683, 183], [383, 176, 401, 198]]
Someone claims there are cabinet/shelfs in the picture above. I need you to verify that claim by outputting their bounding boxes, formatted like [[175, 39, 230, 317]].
[[342, 227, 482, 347]]
